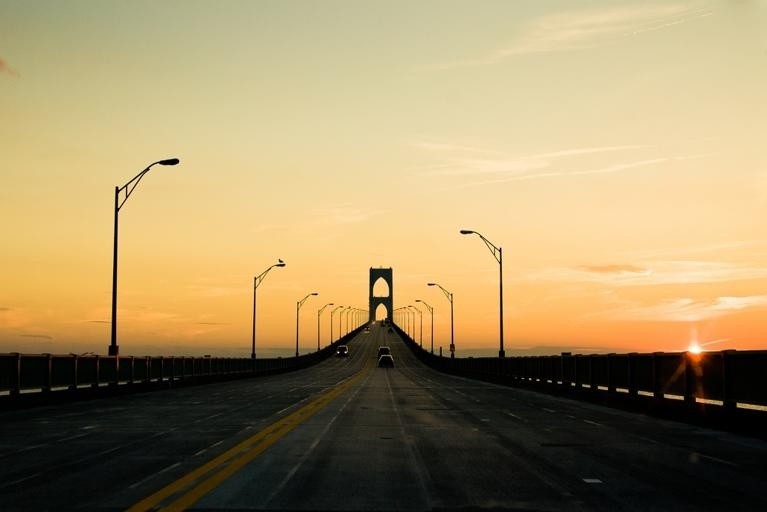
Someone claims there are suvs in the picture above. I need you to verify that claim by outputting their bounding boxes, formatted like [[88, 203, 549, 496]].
[[335, 344, 348, 357], [376, 345, 391, 358]]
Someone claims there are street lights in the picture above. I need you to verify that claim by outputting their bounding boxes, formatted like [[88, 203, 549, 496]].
[[317, 303, 334, 351], [296, 292, 319, 357], [339, 305, 368, 340], [459, 229, 506, 359], [108, 157, 181, 354], [330, 305, 343, 344], [391, 305, 423, 348], [425, 282, 454, 358], [414, 299, 434, 353], [250, 260, 286, 358]]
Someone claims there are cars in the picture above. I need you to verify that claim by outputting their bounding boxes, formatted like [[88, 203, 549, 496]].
[[362, 318, 393, 334], [377, 354, 394, 368]]
